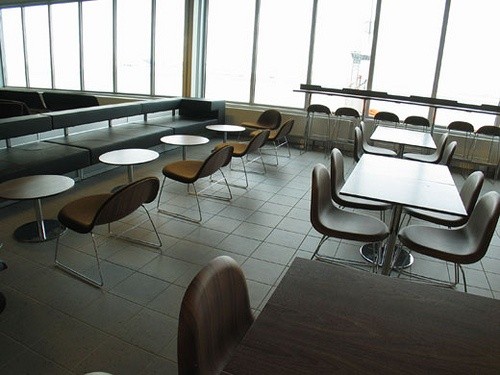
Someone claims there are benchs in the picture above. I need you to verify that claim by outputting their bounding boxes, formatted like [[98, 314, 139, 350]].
[[0, 87, 224, 180]]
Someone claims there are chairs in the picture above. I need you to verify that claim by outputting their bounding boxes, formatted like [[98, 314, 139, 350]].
[[54, 105, 499, 374]]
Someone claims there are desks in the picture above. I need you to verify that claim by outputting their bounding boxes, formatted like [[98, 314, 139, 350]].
[[218, 256, 500, 375], [339, 153, 468, 276], [206, 124, 245, 143], [160, 134, 210, 160], [370, 126, 437, 158], [98, 149, 160, 193], [0, 174, 75, 243]]
